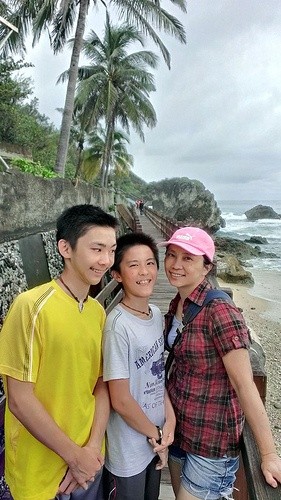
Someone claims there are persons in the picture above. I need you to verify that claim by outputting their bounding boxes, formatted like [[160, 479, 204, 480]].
[[0, 204, 120, 500], [157, 227, 281, 500], [101, 233, 176, 500], [136, 200, 144, 212]]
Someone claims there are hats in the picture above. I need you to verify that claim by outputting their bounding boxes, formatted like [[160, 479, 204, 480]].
[[157, 227, 215, 264]]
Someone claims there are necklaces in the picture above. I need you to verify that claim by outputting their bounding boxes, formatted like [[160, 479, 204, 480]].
[[59, 276, 88, 312], [122, 302, 151, 318]]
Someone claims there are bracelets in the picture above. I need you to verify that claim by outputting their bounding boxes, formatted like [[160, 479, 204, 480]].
[[261, 450, 281, 460], [147, 426, 163, 445]]
